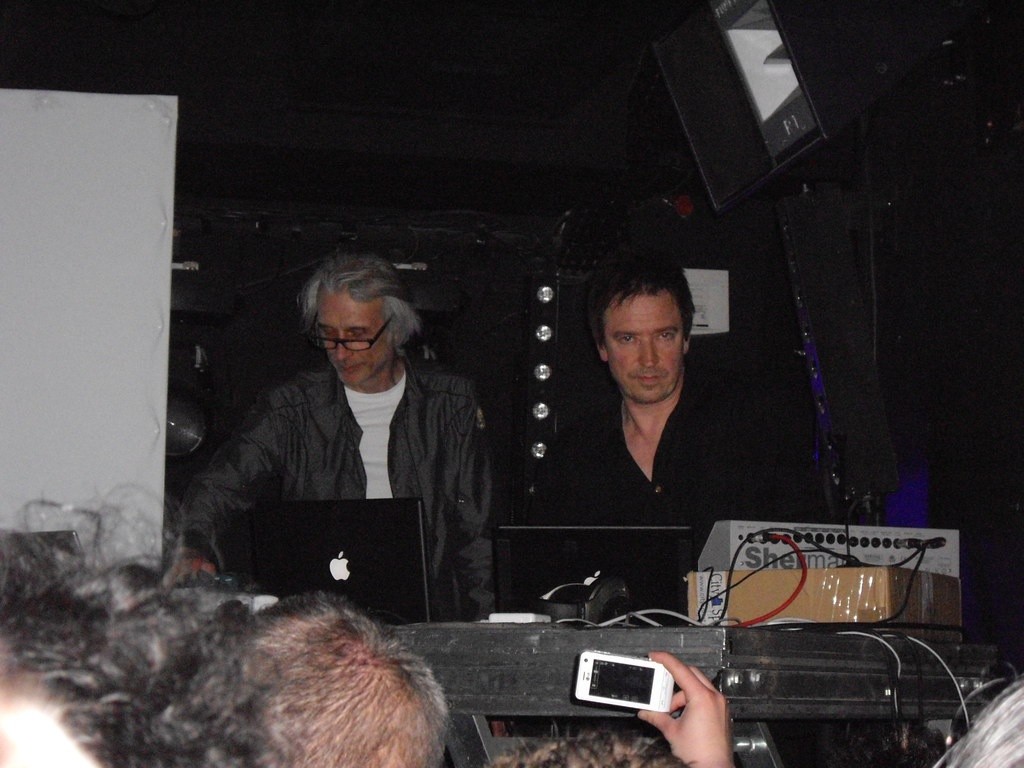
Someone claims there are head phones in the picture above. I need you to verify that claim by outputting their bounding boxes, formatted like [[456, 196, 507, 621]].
[[538, 568, 633, 627]]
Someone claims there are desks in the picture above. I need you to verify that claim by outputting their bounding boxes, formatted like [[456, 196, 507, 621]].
[[381, 619, 996, 768]]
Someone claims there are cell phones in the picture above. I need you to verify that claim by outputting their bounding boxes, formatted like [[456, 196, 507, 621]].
[[575, 650, 674, 713]]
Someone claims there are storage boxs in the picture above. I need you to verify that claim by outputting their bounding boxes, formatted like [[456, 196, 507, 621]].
[[686, 566, 963, 644]]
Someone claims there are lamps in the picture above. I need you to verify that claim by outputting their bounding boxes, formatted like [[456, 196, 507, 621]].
[[648, 0, 984, 218], [166, 394, 211, 459]]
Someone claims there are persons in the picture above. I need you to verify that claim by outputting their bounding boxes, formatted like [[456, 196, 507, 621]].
[[171, 251, 494, 620], [519, 248, 815, 622], [0, 537, 735, 768]]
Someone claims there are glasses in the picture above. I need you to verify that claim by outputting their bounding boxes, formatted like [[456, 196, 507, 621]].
[[306, 313, 396, 351]]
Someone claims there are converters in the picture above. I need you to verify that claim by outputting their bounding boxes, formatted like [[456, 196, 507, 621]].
[[488, 613, 552, 623]]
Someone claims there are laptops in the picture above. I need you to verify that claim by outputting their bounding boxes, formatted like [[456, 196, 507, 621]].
[[251, 497, 433, 627], [493, 526, 694, 622]]
